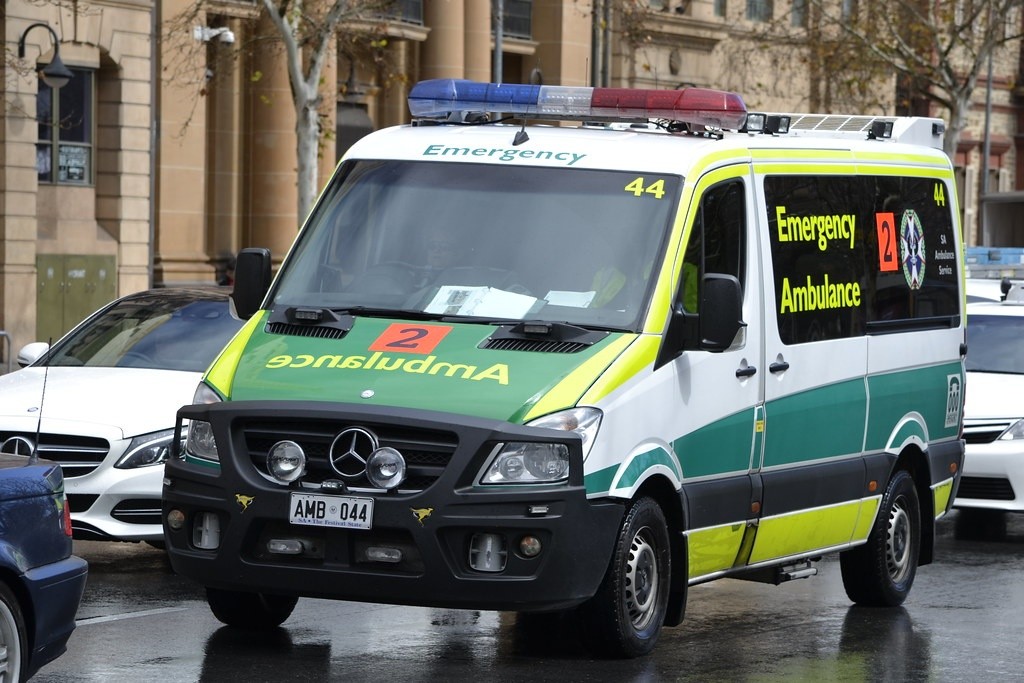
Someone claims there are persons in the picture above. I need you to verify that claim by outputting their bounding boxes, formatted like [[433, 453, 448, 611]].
[[590, 218, 701, 315], [410, 224, 466, 288]]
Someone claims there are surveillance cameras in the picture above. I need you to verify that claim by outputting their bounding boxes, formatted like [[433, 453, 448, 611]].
[[221, 32, 235, 48]]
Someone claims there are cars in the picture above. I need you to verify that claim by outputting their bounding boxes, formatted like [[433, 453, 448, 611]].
[[943, 259, 1024, 529], [0, 448, 89, 683], [0, 282, 267, 548]]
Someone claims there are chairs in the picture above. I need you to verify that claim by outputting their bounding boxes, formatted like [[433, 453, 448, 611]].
[[684, 188, 727, 273]]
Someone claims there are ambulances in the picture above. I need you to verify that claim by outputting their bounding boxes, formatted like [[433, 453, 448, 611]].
[[157, 74, 972, 656]]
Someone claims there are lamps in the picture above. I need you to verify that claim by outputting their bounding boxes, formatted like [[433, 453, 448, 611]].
[[18, 23, 74, 88]]
